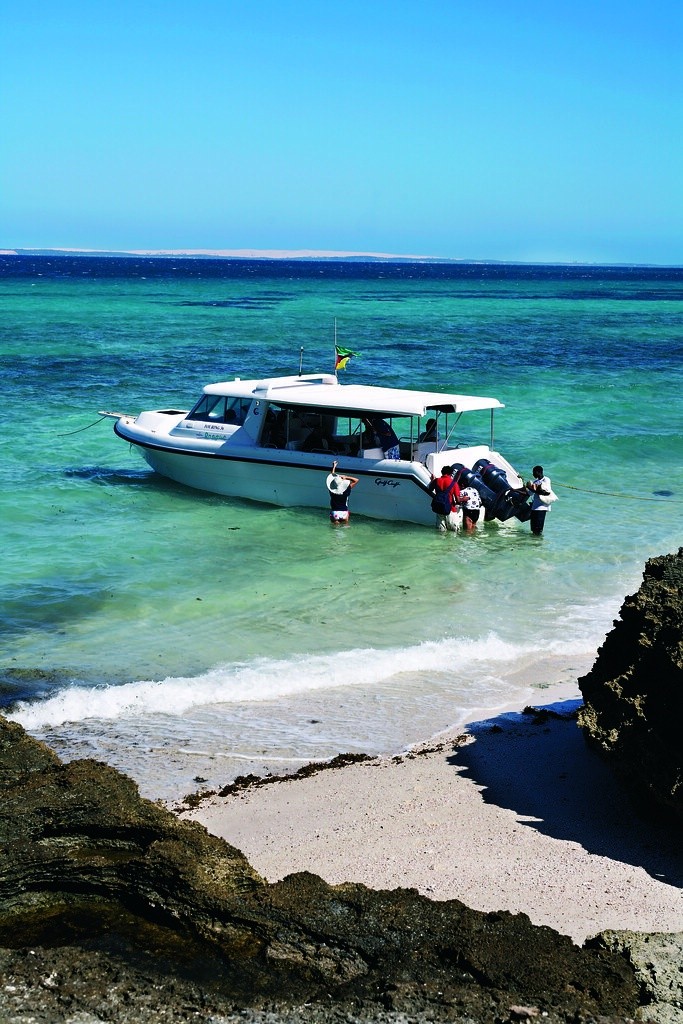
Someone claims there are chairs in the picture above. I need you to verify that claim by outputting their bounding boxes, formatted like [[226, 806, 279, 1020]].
[[359, 447, 385, 460]]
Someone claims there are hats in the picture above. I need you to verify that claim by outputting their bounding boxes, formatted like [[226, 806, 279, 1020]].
[[326, 473, 350, 494]]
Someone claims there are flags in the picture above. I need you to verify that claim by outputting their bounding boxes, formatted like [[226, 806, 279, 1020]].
[[335, 344, 362, 370]]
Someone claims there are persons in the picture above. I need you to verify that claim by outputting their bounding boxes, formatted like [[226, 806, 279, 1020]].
[[416, 419, 442, 442], [430, 466, 469, 530], [457, 479, 482, 532], [526, 466, 554, 537], [225, 407, 382, 458], [327, 461, 359, 526]]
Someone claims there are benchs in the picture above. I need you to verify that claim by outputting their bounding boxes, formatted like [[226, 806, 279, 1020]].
[[412, 439, 448, 463]]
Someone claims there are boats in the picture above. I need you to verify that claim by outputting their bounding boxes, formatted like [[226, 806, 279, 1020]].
[[99, 316, 533, 530]]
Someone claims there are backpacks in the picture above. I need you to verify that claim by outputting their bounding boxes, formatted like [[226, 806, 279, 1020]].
[[431, 480, 455, 516]]
